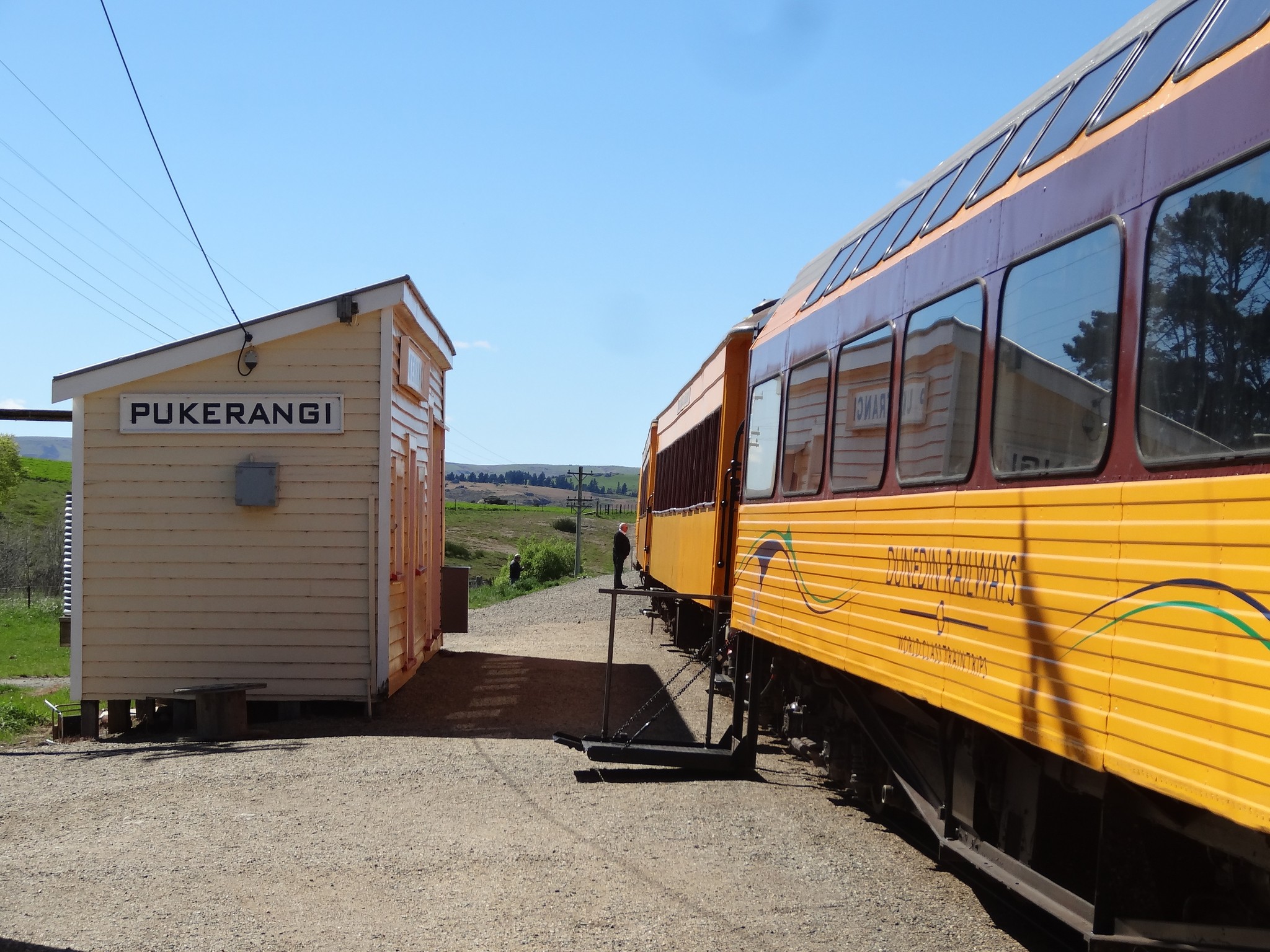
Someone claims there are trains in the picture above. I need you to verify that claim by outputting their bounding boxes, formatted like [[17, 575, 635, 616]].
[[634, 1, 1269, 952]]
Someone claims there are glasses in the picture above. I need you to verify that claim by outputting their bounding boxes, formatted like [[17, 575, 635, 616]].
[[622, 527, 628, 528]]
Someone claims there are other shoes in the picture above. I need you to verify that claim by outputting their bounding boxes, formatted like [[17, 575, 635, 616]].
[[622, 585, 629, 588], [614, 585, 626, 588]]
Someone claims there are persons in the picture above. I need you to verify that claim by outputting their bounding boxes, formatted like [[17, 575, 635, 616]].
[[509, 554, 525, 585], [612, 522, 631, 589]]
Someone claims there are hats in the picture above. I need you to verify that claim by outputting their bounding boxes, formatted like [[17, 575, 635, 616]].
[[515, 554, 523, 558]]
